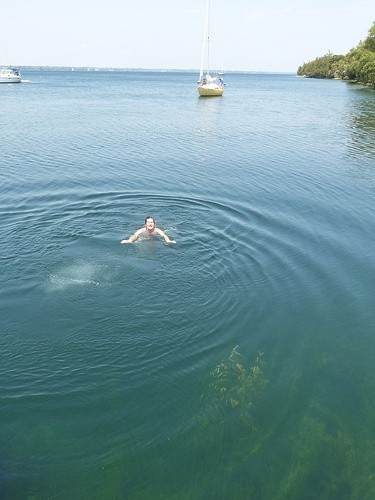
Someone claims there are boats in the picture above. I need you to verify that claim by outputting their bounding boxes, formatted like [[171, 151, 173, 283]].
[[0, 67, 23, 83]]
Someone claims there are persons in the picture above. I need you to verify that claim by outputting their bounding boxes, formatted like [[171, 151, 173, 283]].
[[120, 216, 176, 244]]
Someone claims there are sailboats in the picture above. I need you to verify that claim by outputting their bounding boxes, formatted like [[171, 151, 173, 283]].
[[198, 0, 225, 96]]
